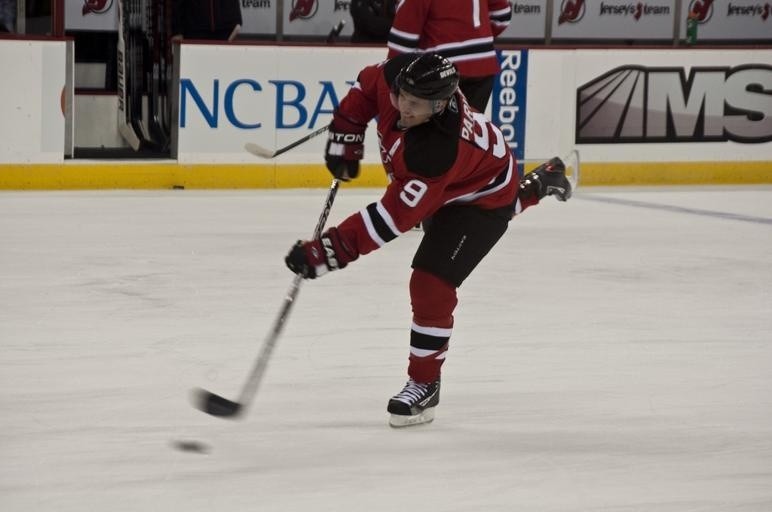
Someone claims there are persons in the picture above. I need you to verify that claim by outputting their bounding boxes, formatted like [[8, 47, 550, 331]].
[[282, 50, 575, 417], [386, 0, 512, 115]]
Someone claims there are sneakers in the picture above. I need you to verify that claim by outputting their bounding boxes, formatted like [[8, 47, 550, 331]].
[[533, 157, 572, 202], [387, 377, 440, 415]]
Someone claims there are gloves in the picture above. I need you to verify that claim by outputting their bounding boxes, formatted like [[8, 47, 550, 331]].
[[324, 123, 364, 182], [285, 230, 341, 280]]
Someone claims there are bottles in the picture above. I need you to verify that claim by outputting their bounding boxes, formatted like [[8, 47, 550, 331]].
[[687, 11, 698, 45]]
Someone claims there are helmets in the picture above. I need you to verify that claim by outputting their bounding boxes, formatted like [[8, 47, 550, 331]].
[[397, 53, 459, 101]]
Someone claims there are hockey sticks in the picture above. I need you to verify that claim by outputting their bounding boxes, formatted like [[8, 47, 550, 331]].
[[188, 177, 340, 423], [117, 0, 171, 153], [244, 123, 330, 159]]
